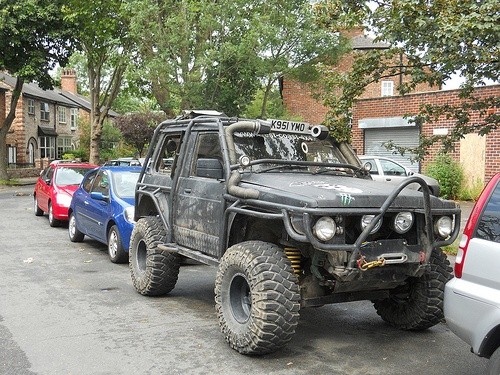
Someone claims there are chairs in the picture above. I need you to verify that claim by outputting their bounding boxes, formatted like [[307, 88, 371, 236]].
[[195, 158, 223, 178]]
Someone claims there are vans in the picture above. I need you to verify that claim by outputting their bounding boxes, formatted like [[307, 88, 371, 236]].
[[319, 156, 441, 199]]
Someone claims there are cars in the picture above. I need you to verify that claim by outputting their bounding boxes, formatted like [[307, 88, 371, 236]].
[[113, 157, 174, 168], [34, 160, 102, 228], [68, 160, 145, 263]]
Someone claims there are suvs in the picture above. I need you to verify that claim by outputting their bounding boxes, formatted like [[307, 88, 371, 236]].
[[128, 109, 463, 355], [443, 172, 500, 375]]
